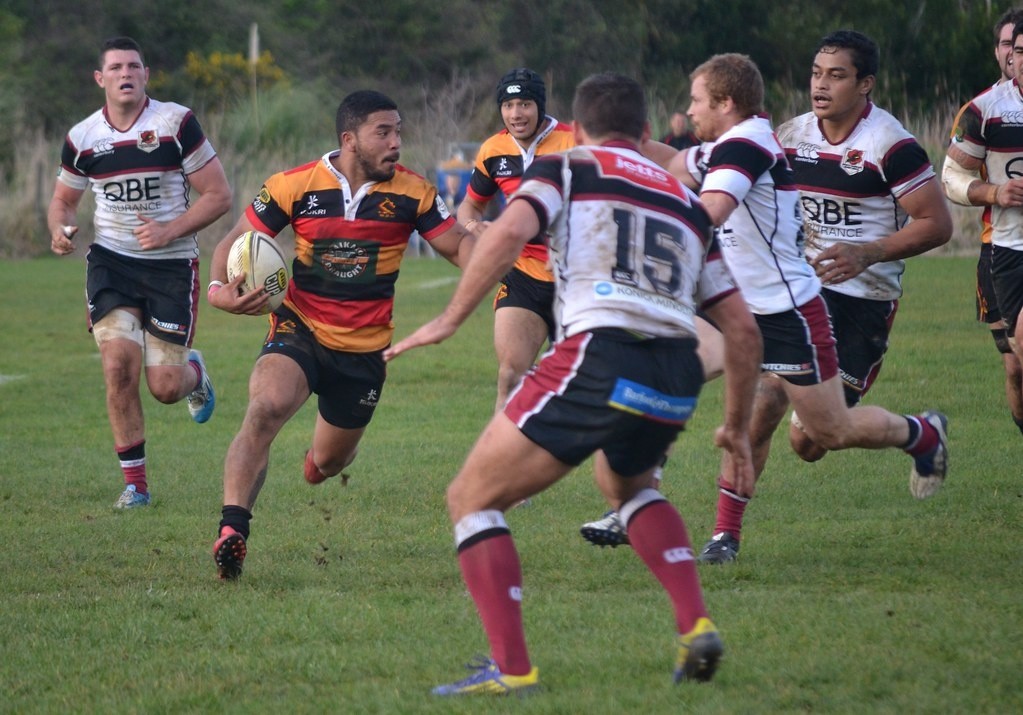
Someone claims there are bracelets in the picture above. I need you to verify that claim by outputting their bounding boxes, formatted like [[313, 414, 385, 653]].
[[208, 280, 225, 290], [987, 185, 999, 205], [463, 219, 475, 228]]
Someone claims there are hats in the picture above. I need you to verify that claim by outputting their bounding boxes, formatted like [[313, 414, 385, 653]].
[[497, 68, 547, 134]]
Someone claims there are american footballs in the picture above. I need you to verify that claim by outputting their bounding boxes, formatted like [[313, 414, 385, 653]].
[[226, 230, 289, 315]]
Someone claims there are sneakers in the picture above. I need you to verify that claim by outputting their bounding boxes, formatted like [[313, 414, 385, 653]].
[[909, 410, 949, 496], [581, 510, 633, 548], [185, 349, 214, 424], [114, 484, 151, 511], [429, 664, 540, 694], [694, 535, 740, 563], [304, 449, 325, 485], [213, 533, 249, 580], [673, 617, 724, 686]]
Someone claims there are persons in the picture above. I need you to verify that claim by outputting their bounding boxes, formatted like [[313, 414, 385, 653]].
[[578, 52, 948, 549], [700, 31, 953, 566], [953, 10, 1023, 434], [206, 90, 475, 580], [48, 38, 232, 507], [941, 20, 1023, 364], [381, 72, 762, 697], [456, 68, 580, 415], [659, 112, 701, 152]]
